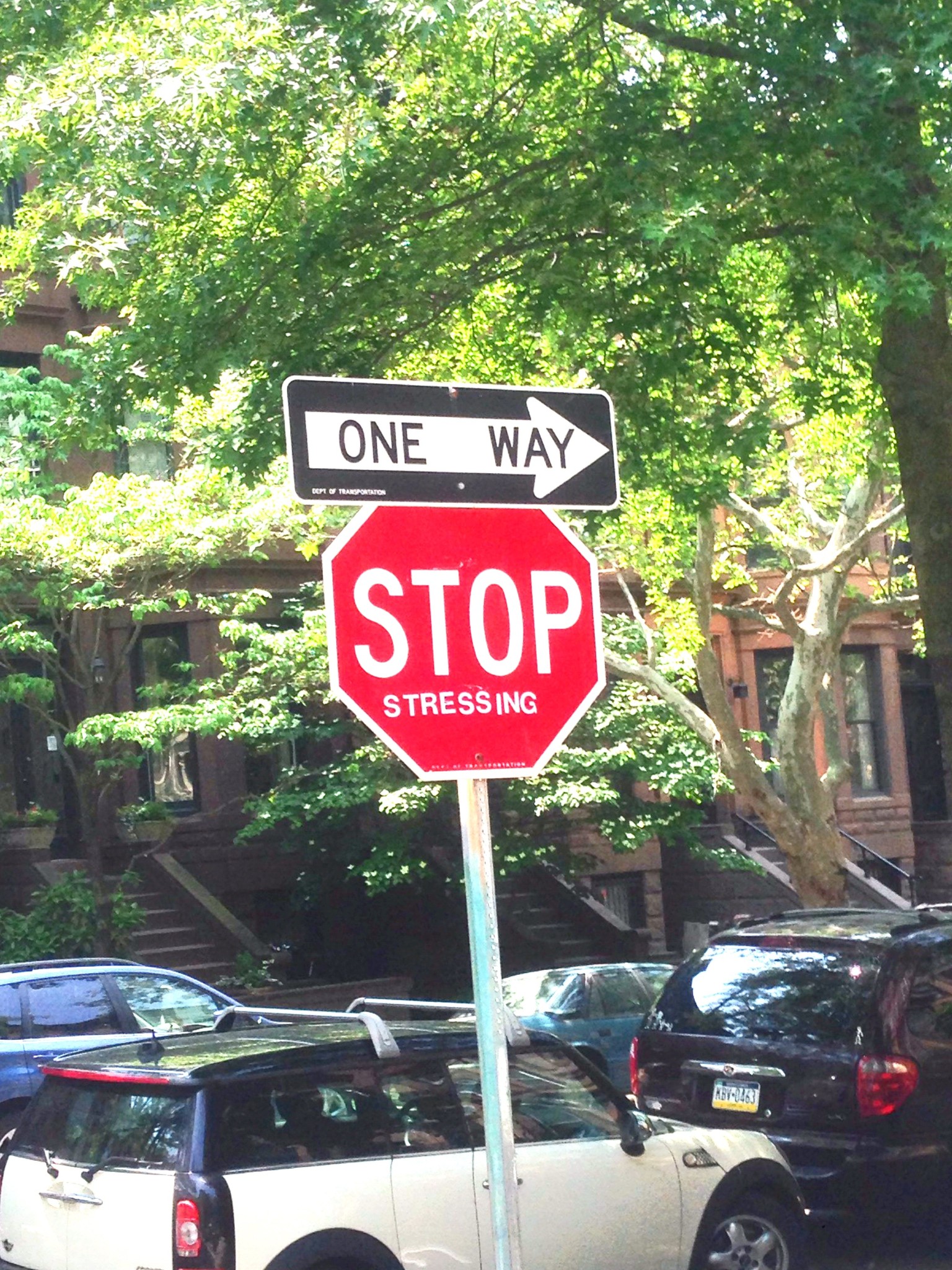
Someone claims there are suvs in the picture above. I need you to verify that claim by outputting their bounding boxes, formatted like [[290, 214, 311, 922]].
[[0, 955, 291, 1175], [628, 907, 952, 1255], [0, 995, 804, 1270]]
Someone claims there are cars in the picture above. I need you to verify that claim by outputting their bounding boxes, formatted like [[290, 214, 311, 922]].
[[444, 963, 682, 1097]]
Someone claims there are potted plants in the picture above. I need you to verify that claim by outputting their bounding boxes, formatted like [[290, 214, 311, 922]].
[[214, 950, 280, 997], [0, 801, 59, 850], [111, 796, 176, 843]]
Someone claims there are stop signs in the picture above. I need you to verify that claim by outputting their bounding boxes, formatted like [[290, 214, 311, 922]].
[[320, 504, 605, 781]]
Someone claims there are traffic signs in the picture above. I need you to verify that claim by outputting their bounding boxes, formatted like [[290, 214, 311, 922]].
[[282, 369, 625, 511]]
